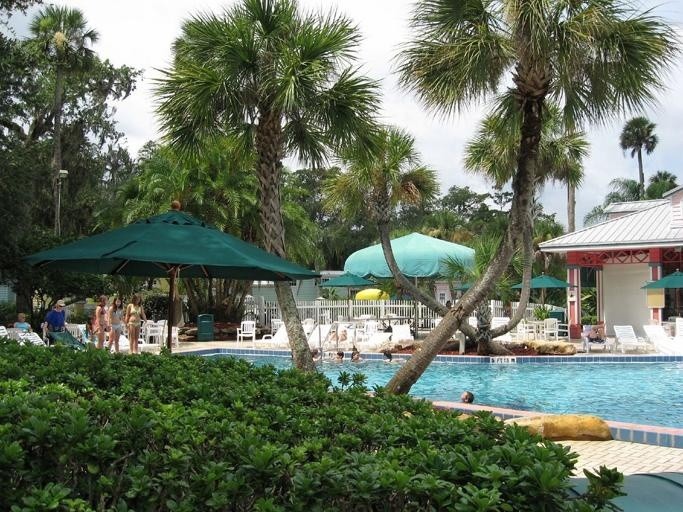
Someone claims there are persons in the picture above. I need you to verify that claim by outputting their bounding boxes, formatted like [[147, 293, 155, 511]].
[[13, 312, 33, 334], [312, 349, 324, 361], [350, 350, 364, 363], [459, 390, 474, 403], [445, 301, 451, 309], [327, 329, 347, 343], [586, 324, 605, 344], [95, 295, 107, 348], [330, 350, 344, 365], [106, 296, 123, 351], [382, 351, 400, 364], [123, 293, 147, 354], [42, 299, 66, 345]]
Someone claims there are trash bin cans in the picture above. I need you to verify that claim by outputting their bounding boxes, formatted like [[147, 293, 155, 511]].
[[197, 314, 214, 342], [548, 309, 566, 336]]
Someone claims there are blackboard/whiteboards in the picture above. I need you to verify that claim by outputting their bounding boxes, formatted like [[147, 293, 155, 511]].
[[645, 281, 665, 308]]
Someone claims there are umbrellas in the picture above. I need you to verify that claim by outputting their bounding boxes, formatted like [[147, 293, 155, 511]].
[[639, 267, 682, 314], [342, 230, 478, 335], [450, 281, 474, 291], [508, 271, 578, 306], [316, 271, 382, 300], [17, 200, 323, 356]]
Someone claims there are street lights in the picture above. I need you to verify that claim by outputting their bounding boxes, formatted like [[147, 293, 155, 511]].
[[58, 170, 69, 236]]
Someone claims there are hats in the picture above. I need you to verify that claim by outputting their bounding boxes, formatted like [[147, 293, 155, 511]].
[[56, 300, 65, 307]]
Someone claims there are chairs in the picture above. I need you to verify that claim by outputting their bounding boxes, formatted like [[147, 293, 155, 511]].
[[0, 319, 180, 350], [236, 321, 256, 344], [261, 314, 570, 346], [581, 316, 683, 351]]
